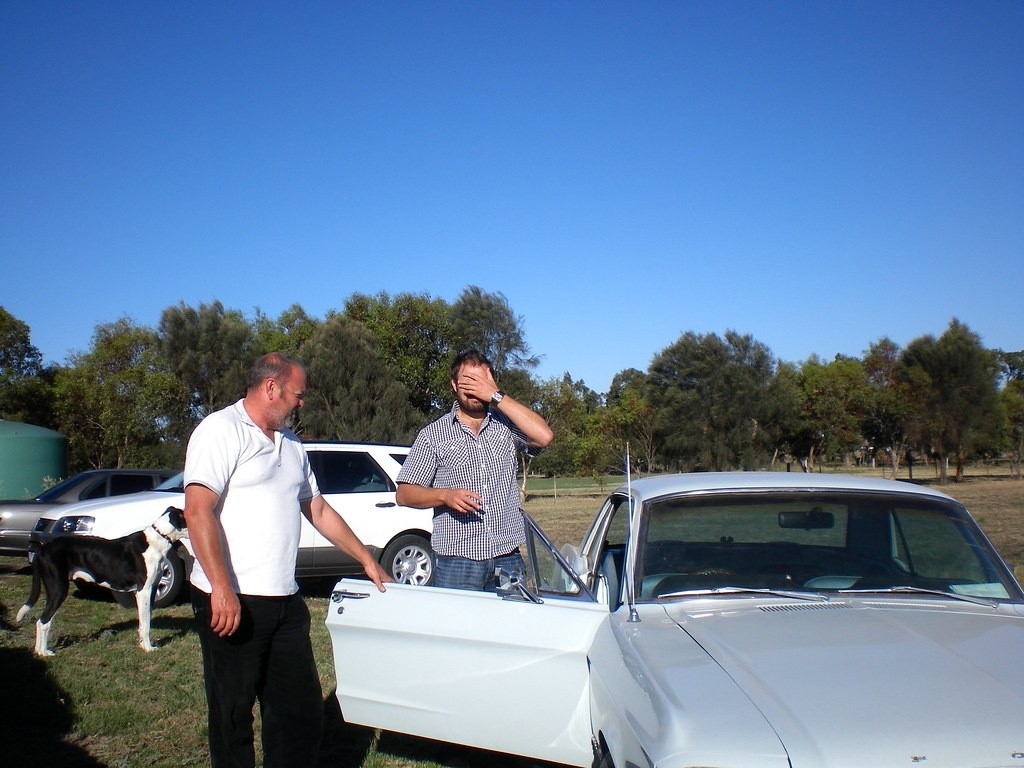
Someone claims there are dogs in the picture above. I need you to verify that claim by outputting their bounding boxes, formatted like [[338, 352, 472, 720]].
[[15, 506, 191, 657]]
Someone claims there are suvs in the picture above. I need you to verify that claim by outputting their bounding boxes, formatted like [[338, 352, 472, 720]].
[[25, 442, 435, 610]]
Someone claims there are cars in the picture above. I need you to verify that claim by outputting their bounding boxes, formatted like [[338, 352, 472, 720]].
[[323, 471, 1023, 768], [0, 467, 182, 557]]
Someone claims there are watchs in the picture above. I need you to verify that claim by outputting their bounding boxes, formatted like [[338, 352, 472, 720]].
[[491, 390, 506, 407]]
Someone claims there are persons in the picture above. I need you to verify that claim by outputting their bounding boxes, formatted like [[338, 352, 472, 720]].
[[183, 353, 398, 768], [394, 350, 554, 595]]
